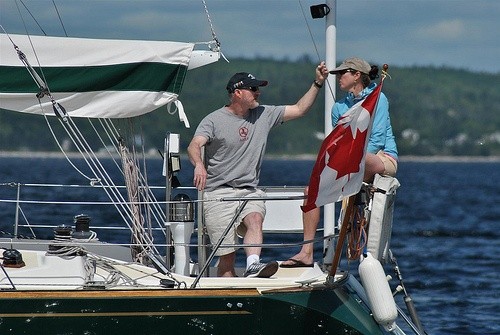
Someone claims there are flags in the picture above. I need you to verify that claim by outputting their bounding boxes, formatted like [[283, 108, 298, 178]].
[[301, 73, 383, 215]]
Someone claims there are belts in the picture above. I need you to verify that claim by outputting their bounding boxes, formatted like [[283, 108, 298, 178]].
[[220, 183, 252, 189]]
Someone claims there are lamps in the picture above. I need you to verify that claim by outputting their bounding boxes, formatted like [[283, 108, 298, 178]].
[[170, 196, 194, 222], [169, 153, 181, 173]]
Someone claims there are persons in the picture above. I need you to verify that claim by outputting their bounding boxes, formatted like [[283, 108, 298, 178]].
[[279, 57, 398, 267], [187, 61, 329, 277]]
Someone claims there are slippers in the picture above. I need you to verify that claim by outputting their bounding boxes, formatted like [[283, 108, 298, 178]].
[[280, 259, 315, 268]]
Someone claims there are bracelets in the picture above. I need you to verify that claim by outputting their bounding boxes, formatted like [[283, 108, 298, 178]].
[[313, 80, 323, 88]]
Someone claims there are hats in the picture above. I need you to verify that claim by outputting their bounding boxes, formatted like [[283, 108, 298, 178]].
[[226, 73, 269, 93], [328, 57, 372, 75]]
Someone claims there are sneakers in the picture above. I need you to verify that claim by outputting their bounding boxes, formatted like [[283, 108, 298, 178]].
[[244, 259, 278, 278]]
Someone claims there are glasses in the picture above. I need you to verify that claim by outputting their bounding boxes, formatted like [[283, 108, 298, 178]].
[[238, 86, 260, 92], [338, 69, 354, 75]]
